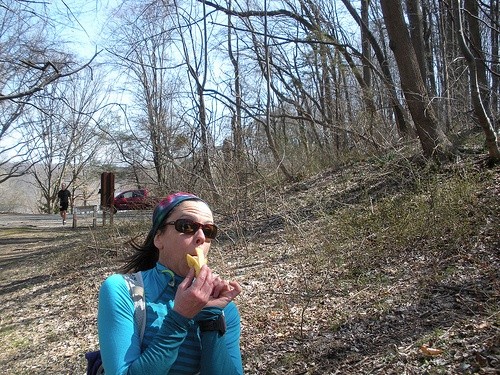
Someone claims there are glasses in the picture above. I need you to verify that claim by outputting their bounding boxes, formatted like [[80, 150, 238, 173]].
[[164, 219, 218, 240]]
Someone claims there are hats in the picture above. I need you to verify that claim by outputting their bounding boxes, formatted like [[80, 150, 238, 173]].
[[152, 192, 210, 237]]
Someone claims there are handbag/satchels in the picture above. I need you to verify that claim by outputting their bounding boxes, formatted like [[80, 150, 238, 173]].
[[86, 270, 146, 375]]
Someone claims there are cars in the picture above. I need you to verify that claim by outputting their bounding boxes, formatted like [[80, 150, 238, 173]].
[[108, 189, 158, 214]]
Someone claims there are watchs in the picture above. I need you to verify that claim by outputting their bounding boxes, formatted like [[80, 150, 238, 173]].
[[198, 315, 227, 337]]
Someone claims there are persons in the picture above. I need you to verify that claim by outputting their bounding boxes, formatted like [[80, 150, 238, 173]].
[[55, 183, 72, 226], [95, 192, 244, 375]]
